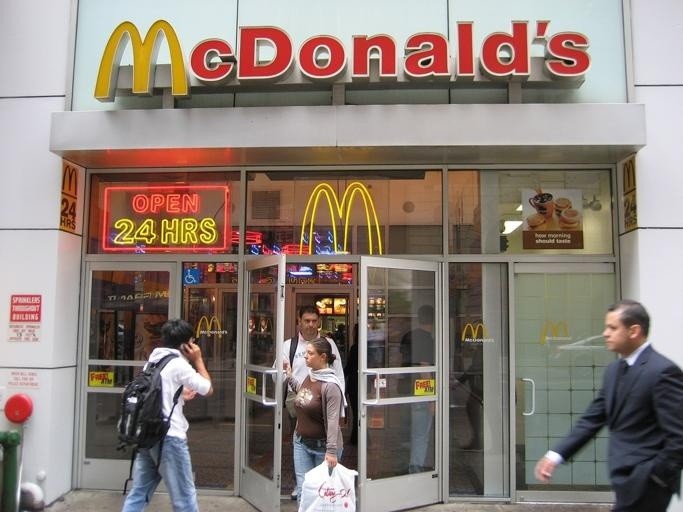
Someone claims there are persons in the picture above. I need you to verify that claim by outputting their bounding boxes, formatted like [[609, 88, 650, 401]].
[[535, 300, 682, 511], [120, 319, 213, 511], [348, 323, 374, 445], [273, 306, 347, 512], [399, 305, 436, 472], [450, 341, 484, 451]]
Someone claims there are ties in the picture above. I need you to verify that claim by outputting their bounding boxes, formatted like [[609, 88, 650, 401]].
[[613, 361, 628, 401]]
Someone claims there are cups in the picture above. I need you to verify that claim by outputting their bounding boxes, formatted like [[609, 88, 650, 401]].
[[529, 192, 554, 217]]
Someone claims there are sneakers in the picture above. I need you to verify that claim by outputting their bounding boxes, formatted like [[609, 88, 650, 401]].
[[392, 448, 426, 474], [290, 487, 300, 500], [457, 434, 483, 452]]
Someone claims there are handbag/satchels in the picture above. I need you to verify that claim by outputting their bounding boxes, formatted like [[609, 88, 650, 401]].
[[284, 392, 298, 418], [298, 461, 359, 512]]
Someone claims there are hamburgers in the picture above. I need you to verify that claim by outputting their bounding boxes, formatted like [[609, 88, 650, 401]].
[[526, 213, 548, 231], [554, 197, 571, 216], [559, 208, 580, 229]]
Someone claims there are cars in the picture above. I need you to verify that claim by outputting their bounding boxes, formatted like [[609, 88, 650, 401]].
[[551, 336, 615, 379], [367, 314, 427, 371]]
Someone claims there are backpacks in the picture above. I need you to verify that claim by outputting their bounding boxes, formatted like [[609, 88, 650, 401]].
[[116, 354, 184, 451]]
